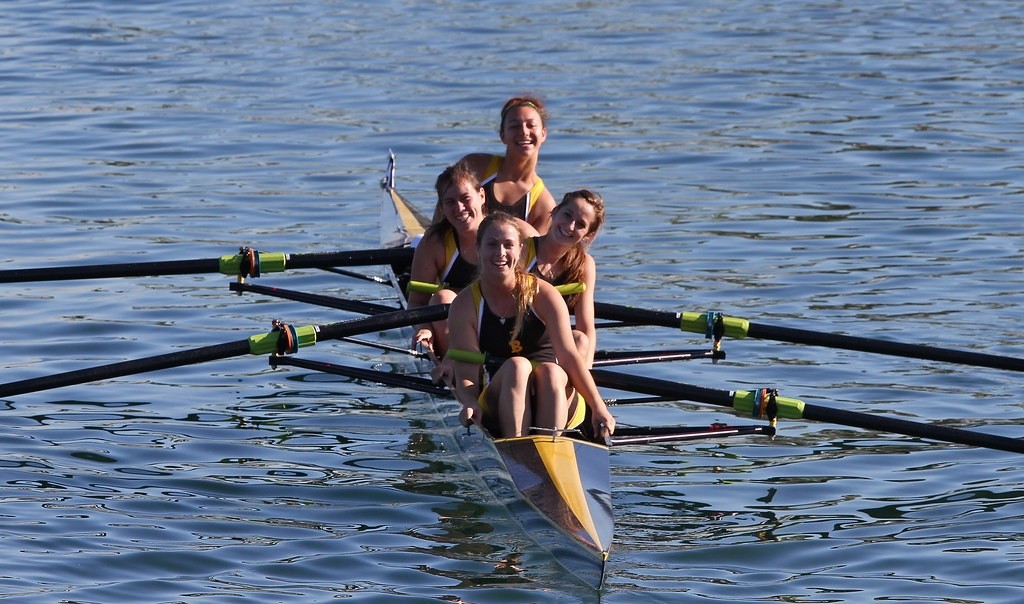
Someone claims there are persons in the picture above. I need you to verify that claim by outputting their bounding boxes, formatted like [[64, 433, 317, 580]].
[[408, 165, 540, 355], [430, 190, 605, 388], [448, 212, 616, 438], [430, 97, 558, 237]]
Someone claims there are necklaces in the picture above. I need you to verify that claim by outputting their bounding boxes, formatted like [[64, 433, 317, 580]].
[[488, 297, 513, 324]]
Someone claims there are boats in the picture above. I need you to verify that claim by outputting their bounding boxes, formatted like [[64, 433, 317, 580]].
[[375, 147, 614, 593]]
